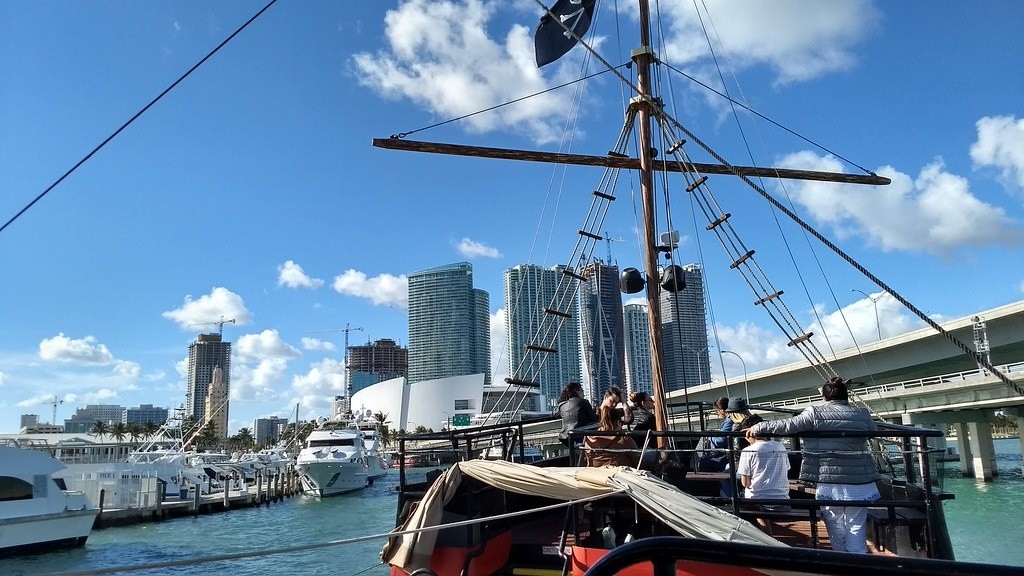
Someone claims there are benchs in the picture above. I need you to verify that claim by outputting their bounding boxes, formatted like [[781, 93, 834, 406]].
[[684, 471, 927, 552]]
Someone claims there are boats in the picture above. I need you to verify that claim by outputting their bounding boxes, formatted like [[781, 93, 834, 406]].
[[1, 438, 102, 558], [54, 410, 388, 498]]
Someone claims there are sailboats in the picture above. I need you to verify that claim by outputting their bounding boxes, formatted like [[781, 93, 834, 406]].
[[370, 2, 1024, 576]]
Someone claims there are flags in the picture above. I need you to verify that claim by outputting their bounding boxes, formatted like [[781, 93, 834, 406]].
[[532, 1, 597, 69]]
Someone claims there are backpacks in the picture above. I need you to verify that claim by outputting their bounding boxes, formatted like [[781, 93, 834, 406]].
[[655, 447, 688, 480]]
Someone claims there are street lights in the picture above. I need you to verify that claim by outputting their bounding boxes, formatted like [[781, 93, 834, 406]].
[[406, 421, 418, 445], [851, 288, 881, 343], [720, 351, 749, 407], [681, 344, 714, 386], [487, 398, 509, 412]]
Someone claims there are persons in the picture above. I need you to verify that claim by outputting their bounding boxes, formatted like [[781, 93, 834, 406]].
[[558, 383, 598, 446], [741, 377, 878, 553], [721, 397, 762, 496], [701, 396, 733, 470], [586, 385, 638, 466], [737, 414, 791, 511], [628, 391, 658, 447]]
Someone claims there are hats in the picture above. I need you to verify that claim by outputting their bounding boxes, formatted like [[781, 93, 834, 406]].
[[725, 397, 749, 412]]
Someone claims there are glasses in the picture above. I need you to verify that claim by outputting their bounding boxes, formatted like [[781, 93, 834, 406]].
[[644, 398, 653, 403]]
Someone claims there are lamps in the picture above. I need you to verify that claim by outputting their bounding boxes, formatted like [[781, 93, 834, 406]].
[[619, 268, 646, 294], [661, 265, 687, 292]]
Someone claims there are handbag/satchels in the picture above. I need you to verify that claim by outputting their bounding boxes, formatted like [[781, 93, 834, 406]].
[[699, 452, 729, 473]]
